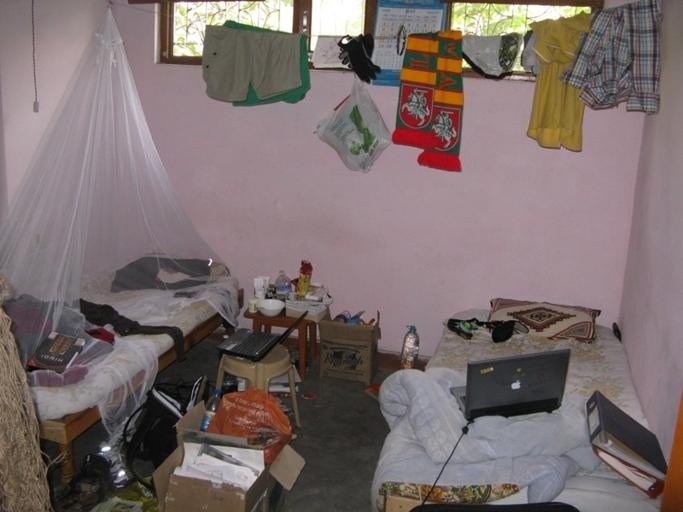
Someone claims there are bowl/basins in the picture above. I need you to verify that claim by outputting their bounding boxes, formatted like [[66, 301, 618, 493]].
[[257, 299, 284, 317]]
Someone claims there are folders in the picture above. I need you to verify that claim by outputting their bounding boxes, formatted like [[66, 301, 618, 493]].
[[587, 391, 668, 497]]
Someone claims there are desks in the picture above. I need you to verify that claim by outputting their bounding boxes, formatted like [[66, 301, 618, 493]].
[[244, 297, 316, 377]]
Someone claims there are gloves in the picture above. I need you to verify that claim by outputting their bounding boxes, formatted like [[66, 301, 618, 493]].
[[337, 32, 381, 84]]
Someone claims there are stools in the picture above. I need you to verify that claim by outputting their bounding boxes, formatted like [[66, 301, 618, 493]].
[[215, 343, 300, 428]]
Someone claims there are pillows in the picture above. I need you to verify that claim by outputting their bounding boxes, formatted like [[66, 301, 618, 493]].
[[115, 256, 229, 290], [487, 298, 600, 343]]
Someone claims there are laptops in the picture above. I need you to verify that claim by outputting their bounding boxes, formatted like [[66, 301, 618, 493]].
[[450, 349, 571, 421], [217, 310, 308, 361]]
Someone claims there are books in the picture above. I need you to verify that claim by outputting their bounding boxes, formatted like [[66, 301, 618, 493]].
[[586, 390, 667, 498], [27, 331, 86, 374], [237, 365, 302, 393], [173, 442, 265, 491]]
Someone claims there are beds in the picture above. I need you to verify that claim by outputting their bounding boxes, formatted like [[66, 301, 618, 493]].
[[371, 309, 663, 511], [0, 257, 243, 483]]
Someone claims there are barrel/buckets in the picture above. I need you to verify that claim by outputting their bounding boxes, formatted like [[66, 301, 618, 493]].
[[397, 324, 420, 369]]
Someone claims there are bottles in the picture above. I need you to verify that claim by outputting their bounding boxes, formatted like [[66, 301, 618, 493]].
[[400, 324, 419, 370], [296, 258, 312, 299], [275, 269, 293, 300], [200, 390, 223, 432]]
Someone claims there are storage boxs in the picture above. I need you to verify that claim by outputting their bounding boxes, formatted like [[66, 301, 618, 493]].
[[152, 399, 305, 511], [318, 319, 381, 386]]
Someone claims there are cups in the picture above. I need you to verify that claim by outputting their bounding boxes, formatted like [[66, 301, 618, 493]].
[[248, 298, 259, 313]]
